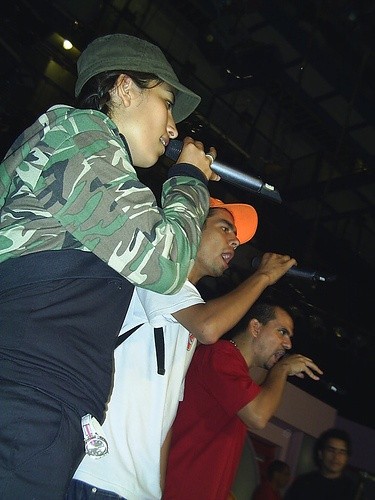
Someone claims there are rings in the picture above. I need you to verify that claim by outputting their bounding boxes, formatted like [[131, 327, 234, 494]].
[[205, 152, 215, 164]]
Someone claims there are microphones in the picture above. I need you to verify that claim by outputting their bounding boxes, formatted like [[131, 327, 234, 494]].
[[252, 255, 325, 281], [164, 140, 282, 202], [301, 366, 337, 391]]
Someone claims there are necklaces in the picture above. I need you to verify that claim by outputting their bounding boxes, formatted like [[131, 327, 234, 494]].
[[229, 339, 238, 347]]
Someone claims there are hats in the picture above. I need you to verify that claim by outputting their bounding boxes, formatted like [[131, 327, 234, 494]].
[[209, 197, 258, 245], [75, 34, 202, 124]]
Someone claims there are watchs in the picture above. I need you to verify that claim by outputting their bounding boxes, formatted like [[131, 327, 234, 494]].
[[81, 413, 112, 461]]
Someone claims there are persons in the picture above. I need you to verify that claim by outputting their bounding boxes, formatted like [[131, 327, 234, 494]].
[[284, 428, 375, 500], [0, 34, 220, 500], [162, 301, 323, 500], [250, 459, 290, 500], [65, 197, 298, 500]]
